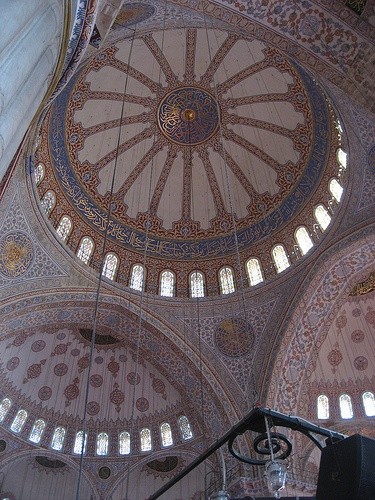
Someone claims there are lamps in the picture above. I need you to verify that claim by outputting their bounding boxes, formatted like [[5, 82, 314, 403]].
[[263, 415, 290, 499]]
